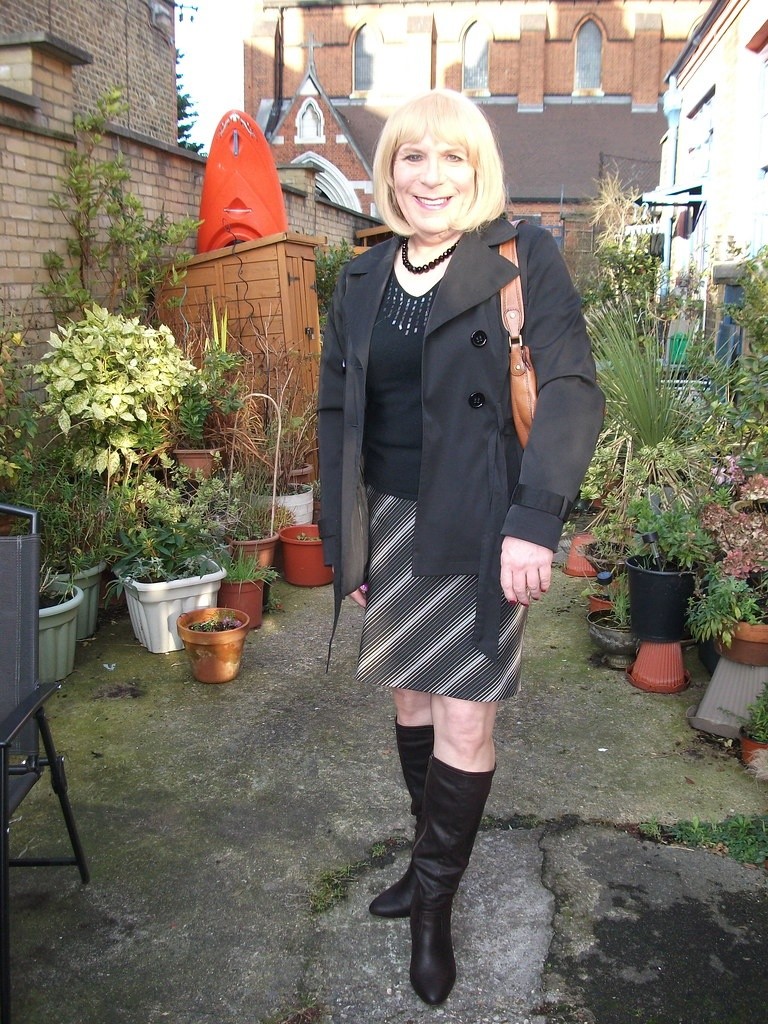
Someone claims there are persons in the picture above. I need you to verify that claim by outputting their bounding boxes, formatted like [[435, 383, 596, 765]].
[[315, 89, 607, 1007]]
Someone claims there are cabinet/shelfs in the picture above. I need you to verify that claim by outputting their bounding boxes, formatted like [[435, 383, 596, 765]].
[[155, 235, 325, 478]]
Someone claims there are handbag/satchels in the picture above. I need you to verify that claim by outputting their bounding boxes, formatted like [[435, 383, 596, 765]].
[[499, 219, 536, 449]]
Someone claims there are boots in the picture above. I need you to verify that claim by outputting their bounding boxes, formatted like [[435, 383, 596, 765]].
[[367, 717, 434, 917], [410, 754, 498, 1008]]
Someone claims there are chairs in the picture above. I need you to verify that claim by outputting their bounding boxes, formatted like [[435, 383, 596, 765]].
[[0, 504, 91, 1024]]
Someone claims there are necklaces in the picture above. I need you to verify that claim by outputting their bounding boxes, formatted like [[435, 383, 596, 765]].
[[402, 238, 460, 274]]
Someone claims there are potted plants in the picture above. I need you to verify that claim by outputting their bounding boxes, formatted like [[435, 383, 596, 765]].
[[581, 287, 768, 675], [739, 681, 768, 765], [0, 81, 333, 684]]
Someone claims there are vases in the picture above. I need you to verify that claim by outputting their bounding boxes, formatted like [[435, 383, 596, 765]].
[[627, 641, 693, 695], [684, 655, 768, 738], [564, 532, 601, 577]]
